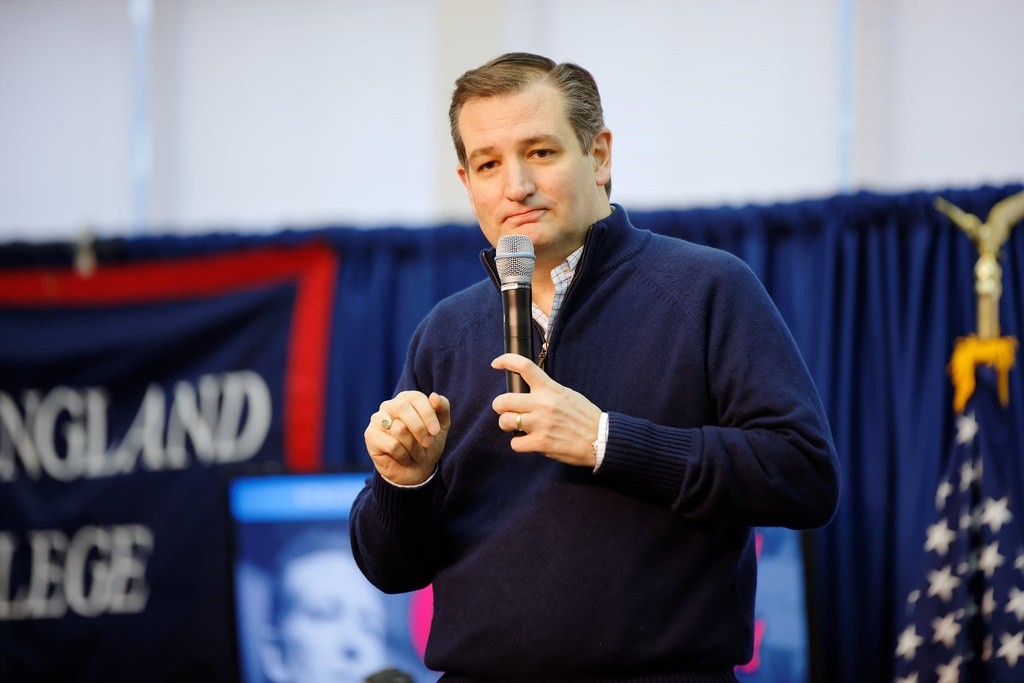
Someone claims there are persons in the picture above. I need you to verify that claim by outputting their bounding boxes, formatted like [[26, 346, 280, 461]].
[[348, 52, 843, 683], [257, 526, 386, 683]]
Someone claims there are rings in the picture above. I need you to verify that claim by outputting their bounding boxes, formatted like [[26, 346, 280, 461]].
[[516, 412, 524, 432], [381, 416, 397, 435]]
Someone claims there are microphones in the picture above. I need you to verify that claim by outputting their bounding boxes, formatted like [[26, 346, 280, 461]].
[[494, 234, 536, 395]]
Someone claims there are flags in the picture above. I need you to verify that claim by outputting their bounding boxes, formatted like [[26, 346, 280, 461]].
[[897, 364, 1024, 683]]
